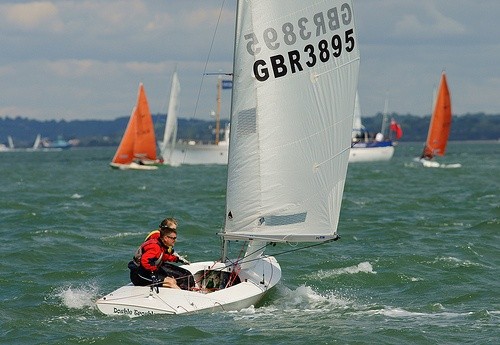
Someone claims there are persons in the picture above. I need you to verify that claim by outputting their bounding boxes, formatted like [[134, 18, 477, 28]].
[[129, 218, 194, 290]]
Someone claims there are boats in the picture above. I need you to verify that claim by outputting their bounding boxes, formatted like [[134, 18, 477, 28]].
[[161, 68, 402, 166], [0, 135, 73, 153]]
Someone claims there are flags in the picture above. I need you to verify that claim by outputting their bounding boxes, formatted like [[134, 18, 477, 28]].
[[391, 118, 403, 138]]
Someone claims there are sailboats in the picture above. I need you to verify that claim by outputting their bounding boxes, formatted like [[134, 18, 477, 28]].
[[413, 68, 462, 170], [107, 82, 166, 170], [94, 0, 359, 314]]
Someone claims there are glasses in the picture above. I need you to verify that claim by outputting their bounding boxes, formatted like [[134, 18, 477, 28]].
[[167, 236, 176, 240]]
